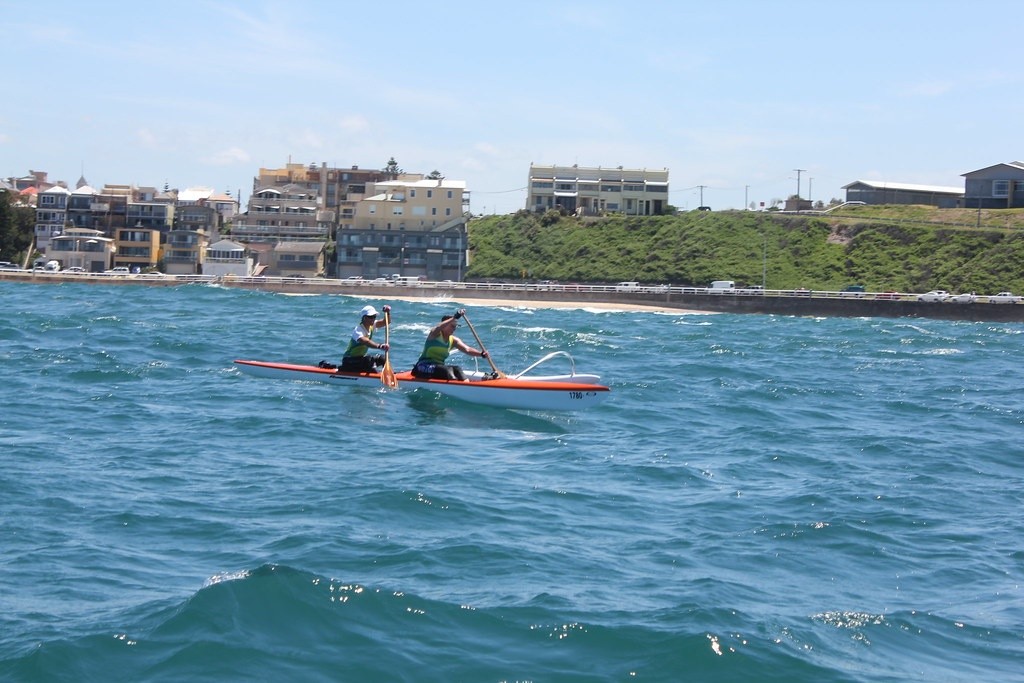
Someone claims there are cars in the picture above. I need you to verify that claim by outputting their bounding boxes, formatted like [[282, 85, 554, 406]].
[[744, 286, 763, 295], [951, 293, 975, 303], [279, 274, 467, 289], [875, 290, 901, 300], [0, 260, 162, 276], [989, 291, 1022, 304], [916, 290, 950, 301]]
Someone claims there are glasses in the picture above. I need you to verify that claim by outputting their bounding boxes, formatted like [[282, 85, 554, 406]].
[[366, 315, 376, 319]]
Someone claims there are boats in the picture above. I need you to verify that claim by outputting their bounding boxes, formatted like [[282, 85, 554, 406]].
[[232, 358, 612, 413]]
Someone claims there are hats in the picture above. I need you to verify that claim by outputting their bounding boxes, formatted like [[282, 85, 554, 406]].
[[361, 305, 380, 318], [442, 315, 462, 327]]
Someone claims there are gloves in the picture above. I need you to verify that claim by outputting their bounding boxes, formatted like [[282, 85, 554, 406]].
[[382, 305, 391, 315], [377, 343, 390, 351]]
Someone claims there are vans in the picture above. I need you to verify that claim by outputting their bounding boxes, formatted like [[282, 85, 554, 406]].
[[839, 286, 864, 298], [704, 280, 735, 295], [615, 282, 640, 292]]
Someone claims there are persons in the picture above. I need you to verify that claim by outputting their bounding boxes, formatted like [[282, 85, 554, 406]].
[[410, 308, 490, 383], [341, 303, 392, 376]]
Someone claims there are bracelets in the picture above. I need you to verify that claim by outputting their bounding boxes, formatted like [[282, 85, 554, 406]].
[[481, 352, 487, 359]]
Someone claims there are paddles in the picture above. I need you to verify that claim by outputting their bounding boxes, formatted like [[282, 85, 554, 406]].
[[463, 313, 507, 379], [381, 311, 398, 389]]
